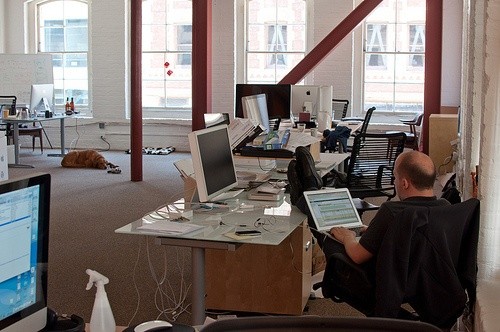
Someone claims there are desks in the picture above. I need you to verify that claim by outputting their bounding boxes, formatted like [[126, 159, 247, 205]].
[[320, 120, 363, 171], [115, 153, 351, 326], [0, 112, 79, 169]]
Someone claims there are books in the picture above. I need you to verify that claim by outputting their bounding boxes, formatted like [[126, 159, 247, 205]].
[[230, 118, 267, 153]]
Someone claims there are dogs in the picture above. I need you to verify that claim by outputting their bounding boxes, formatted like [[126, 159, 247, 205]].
[[61, 150, 109, 170]]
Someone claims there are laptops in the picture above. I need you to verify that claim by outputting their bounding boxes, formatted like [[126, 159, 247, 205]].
[[304, 188, 368, 245]]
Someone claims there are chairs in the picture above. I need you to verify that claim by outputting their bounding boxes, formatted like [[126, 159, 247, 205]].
[[7, 107, 43, 152], [310, 98, 480, 331]]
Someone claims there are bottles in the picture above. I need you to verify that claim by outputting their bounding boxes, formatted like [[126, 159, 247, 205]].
[[66, 97, 71, 115], [70, 98, 74, 114]]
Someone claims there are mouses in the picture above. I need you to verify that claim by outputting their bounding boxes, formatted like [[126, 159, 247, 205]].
[[135, 321, 172, 332]]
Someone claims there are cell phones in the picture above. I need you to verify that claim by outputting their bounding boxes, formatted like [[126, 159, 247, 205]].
[[235, 230, 261, 236]]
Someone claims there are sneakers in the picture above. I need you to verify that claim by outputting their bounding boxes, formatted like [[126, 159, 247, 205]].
[[296, 146, 323, 190], [287, 159, 303, 206]]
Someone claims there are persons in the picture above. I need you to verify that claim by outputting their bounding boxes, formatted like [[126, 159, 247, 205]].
[[287, 146, 453, 300]]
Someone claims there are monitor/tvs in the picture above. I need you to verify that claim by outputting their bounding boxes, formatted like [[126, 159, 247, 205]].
[[188, 124, 239, 211], [30, 83, 54, 112], [235, 84, 333, 121], [0, 172, 52, 332]]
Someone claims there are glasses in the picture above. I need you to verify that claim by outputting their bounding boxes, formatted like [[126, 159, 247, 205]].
[[254, 216, 276, 232]]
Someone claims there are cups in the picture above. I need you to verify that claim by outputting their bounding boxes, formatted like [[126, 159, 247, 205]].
[[3, 110, 9, 118], [311, 128, 319, 137], [297, 123, 306, 133]]
